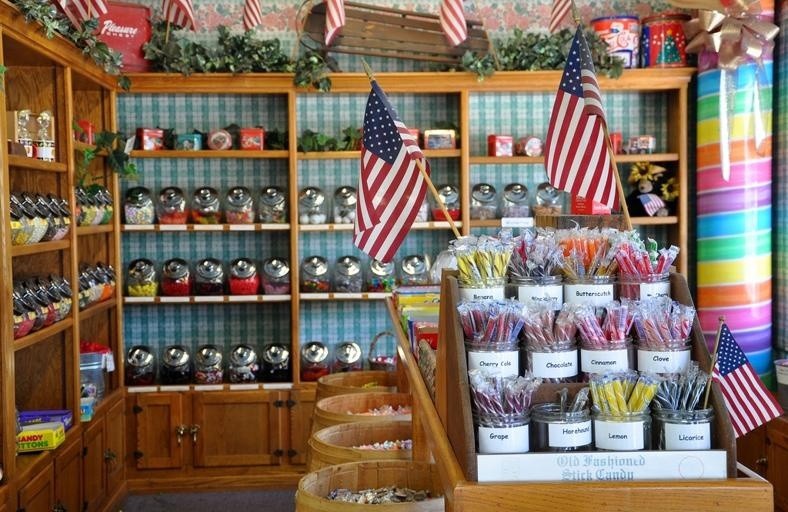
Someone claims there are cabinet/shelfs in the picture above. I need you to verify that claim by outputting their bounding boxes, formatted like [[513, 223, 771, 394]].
[[118, 70, 692, 493], [0, 0, 125, 512], [386, 238, 781, 512]]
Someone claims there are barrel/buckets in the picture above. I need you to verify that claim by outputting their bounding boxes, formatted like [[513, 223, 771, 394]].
[[310, 391, 414, 430], [295, 459, 445, 512], [306, 422, 413, 474], [315, 371, 399, 398]]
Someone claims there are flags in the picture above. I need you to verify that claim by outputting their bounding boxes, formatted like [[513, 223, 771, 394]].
[[323, 1, 345, 48], [549, 0, 576, 34], [353, 80, 430, 265], [162, 1, 197, 34], [711, 324, 784, 438], [58, 0, 110, 33], [639, 194, 665, 217], [544, 24, 621, 212], [243, 0, 263, 34], [438, 0, 468, 50]]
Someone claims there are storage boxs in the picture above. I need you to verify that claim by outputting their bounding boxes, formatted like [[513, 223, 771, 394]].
[[97, 1, 149, 71]]
